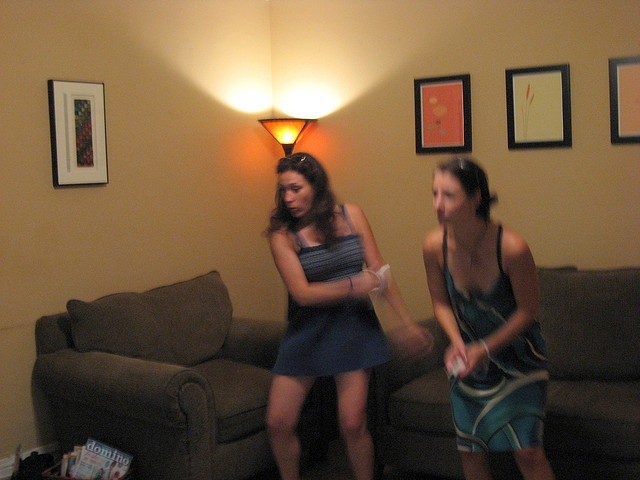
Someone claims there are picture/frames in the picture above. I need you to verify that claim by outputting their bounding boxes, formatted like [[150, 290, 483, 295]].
[[48, 78, 110, 188], [413, 73, 473, 154], [505, 63, 573, 149], [608, 54, 640, 143]]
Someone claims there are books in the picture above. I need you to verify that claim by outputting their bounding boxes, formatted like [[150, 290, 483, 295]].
[[62, 437, 133, 480]]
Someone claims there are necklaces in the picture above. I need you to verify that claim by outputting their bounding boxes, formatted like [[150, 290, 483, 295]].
[[452, 217, 487, 269]]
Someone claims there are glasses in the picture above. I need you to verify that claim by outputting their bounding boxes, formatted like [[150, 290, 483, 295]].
[[279, 156, 314, 173]]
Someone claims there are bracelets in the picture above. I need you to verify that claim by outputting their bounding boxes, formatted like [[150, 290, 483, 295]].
[[346, 274, 354, 297]]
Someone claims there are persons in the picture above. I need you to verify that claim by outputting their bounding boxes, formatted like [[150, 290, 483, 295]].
[[421, 155, 556, 480], [261, 152, 435, 479]]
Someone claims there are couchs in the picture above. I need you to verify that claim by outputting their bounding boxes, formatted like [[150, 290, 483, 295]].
[[368, 264, 640, 480], [33, 270, 289, 478]]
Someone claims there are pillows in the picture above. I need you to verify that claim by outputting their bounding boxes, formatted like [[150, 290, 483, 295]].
[[66, 270, 233, 366], [533, 266, 640, 381]]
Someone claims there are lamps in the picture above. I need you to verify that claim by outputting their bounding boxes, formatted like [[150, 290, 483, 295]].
[[258, 118, 316, 155]]
